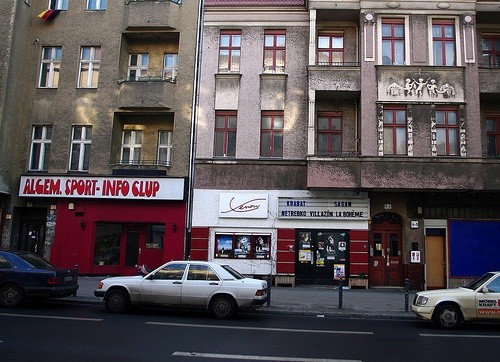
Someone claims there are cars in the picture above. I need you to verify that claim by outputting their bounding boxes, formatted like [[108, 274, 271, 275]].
[[0, 250, 79, 308], [410, 271, 500, 330], [94, 261, 268, 319]]
